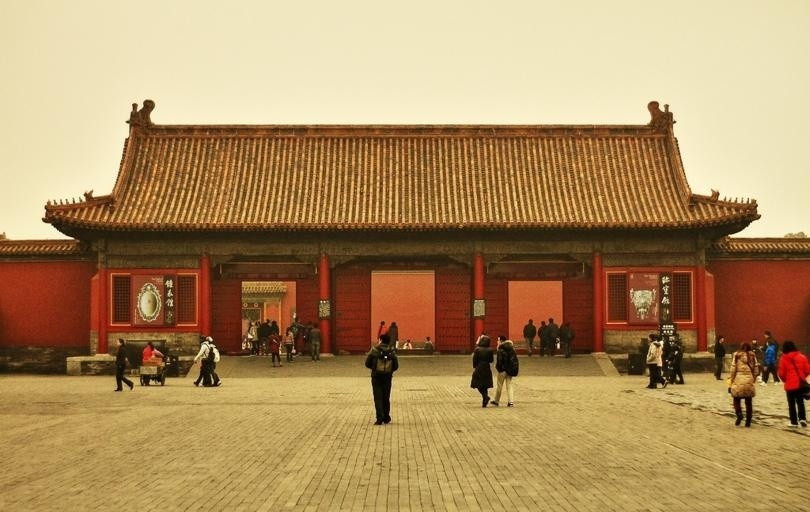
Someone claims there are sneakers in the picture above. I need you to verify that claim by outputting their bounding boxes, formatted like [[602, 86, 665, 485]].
[[114, 384, 134, 391], [375, 417, 391, 424], [482, 396, 498, 407], [788, 420, 807, 428], [648, 380, 684, 389], [508, 403, 514, 407], [194, 380, 221, 387], [774, 381, 779, 385], [736, 416, 751, 428], [760, 381, 767, 386]]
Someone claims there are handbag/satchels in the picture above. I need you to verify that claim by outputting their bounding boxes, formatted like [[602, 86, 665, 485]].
[[799, 377, 810, 400], [291, 350, 296, 354], [272, 339, 278, 344]]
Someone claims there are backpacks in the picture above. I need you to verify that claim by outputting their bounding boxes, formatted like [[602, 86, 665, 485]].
[[499, 346, 519, 376], [373, 347, 395, 374], [202, 343, 220, 363]]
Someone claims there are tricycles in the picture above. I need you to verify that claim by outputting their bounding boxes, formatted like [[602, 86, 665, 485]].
[[137, 353, 168, 388]]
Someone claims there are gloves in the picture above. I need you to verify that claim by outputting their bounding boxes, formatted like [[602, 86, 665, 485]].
[[728, 388, 731, 393]]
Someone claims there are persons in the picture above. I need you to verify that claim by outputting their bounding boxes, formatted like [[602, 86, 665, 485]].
[[113, 338, 133, 391], [194, 333, 222, 387], [403, 339, 412, 350], [471, 335, 494, 407], [142, 342, 164, 386], [523, 319, 575, 358], [378, 320, 399, 350], [423, 336, 434, 349], [751, 331, 780, 385], [779, 339, 810, 428], [645, 333, 684, 389], [244, 317, 321, 367], [490, 336, 517, 408], [728, 342, 759, 426], [365, 334, 398, 424], [714, 335, 725, 380]]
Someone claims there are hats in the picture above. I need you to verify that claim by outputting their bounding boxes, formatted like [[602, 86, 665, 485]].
[[206, 336, 213, 342]]
